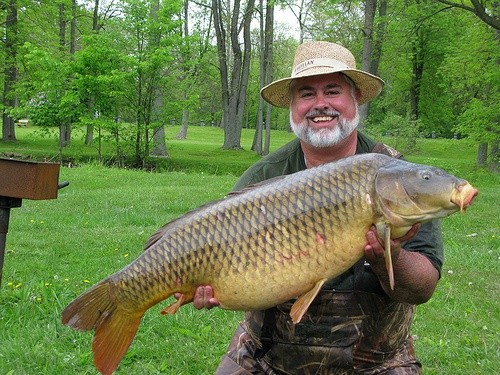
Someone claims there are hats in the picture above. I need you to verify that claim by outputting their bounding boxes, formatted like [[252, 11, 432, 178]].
[[259, 41, 386, 110]]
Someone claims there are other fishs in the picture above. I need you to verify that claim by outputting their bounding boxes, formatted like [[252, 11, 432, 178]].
[[63, 151, 479, 374]]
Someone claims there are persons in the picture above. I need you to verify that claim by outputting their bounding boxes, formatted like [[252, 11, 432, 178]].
[[175, 41, 443, 374]]
